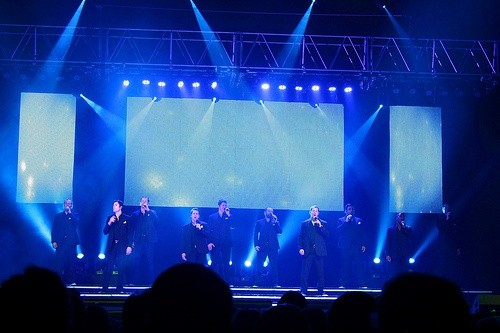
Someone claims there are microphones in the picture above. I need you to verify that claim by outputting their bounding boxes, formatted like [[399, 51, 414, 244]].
[[196, 219, 199, 224], [66, 208, 69, 211], [224, 208, 229, 211], [315, 217, 319, 226]]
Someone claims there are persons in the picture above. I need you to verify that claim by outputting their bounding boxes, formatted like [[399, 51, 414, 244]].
[[98, 200, 132, 294], [299, 205, 329, 297], [335, 204, 368, 289], [207, 200, 234, 287], [180, 207, 209, 266], [384, 213, 413, 274], [252, 206, 282, 289], [1, 263, 500, 333], [51, 198, 81, 286], [436, 204, 453, 233], [127, 197, 159, 287]]
